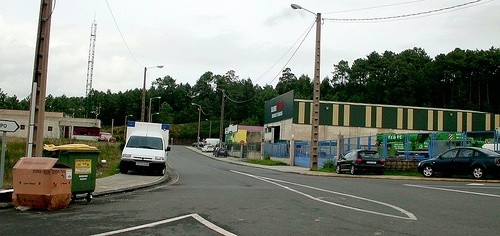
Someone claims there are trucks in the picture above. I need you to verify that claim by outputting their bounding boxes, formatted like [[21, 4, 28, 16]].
[[120, 121, 171, 176]]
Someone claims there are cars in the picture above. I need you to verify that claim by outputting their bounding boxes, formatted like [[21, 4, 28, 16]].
[[417, 146, 500, 179], [192, 138, 228, 157], [336, 149, 385, 175]]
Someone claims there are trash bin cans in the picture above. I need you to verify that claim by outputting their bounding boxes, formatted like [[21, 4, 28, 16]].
[[42, 144, 101, 204]]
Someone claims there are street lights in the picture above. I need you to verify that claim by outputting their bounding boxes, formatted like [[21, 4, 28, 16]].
[[148, 97, 161, 123], [192, 103, 201, 148], [206, 119, 211, 138], [141, 65, 163, 123], [291, 3, 321, 171]]
